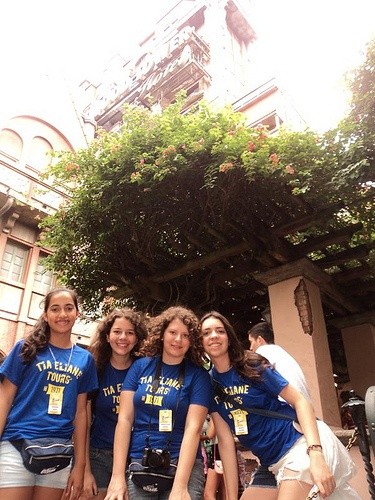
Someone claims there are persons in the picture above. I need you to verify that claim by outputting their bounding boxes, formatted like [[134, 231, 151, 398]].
[[86, 306, 148, 500], [198, 414, 228, 500], [247, 322, 311, 413], [197, 311, 356, 500], [0, 289, 97, 500], [103, 304, 212, 500]]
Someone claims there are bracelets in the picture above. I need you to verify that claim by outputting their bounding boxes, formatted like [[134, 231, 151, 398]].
[[307, 444, 323, 455]]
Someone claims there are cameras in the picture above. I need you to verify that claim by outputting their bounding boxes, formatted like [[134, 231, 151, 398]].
[[142, 448, 171, 469]]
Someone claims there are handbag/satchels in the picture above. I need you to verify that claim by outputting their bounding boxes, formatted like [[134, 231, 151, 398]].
[[213, 460, 223, 474], [10, 437, 74, 475], [125, 461, 178, 494]]
